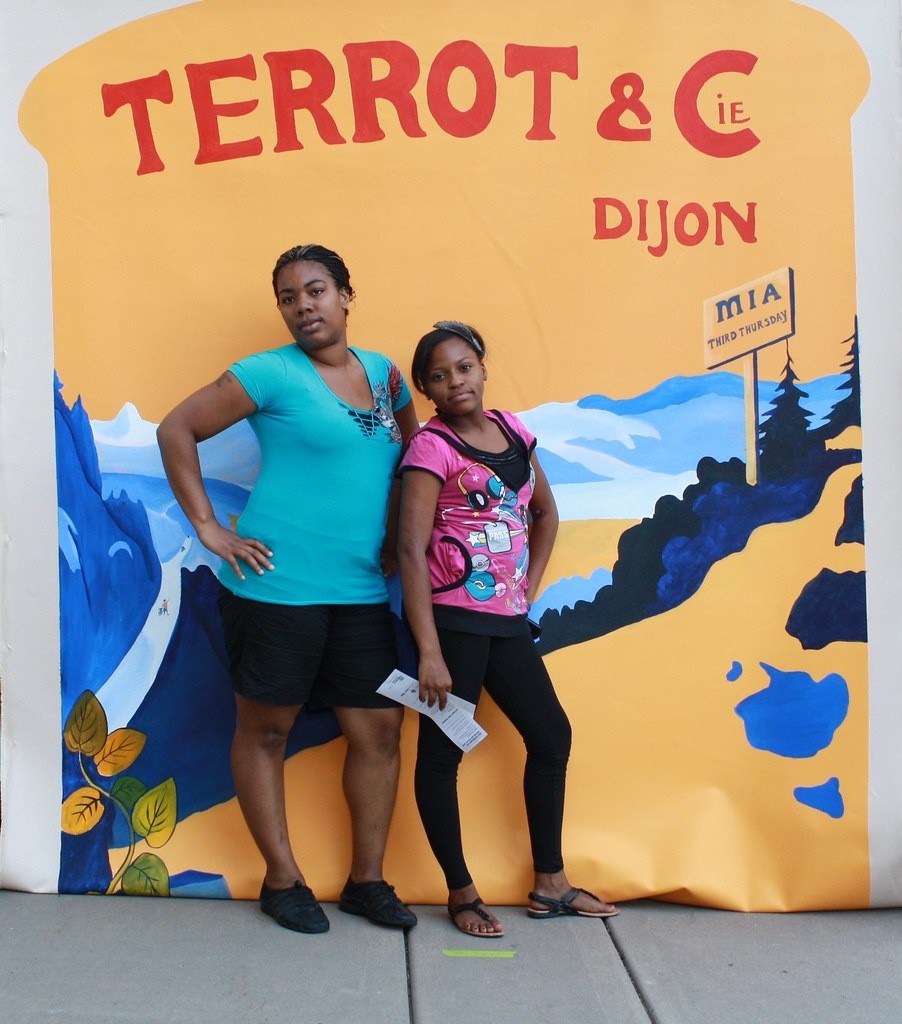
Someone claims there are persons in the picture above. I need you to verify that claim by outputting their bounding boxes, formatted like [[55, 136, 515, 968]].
[[396, 319, 621, 938], [157, 244, 419, 934]]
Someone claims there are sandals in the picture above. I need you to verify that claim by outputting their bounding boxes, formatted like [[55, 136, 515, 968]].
[[447, 898, 504, 936], [527, 881, 621, 919]]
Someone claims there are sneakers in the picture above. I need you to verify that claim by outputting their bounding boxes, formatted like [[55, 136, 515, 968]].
[[259, 876, 330, 933], [338, 874, 418, 928]]
